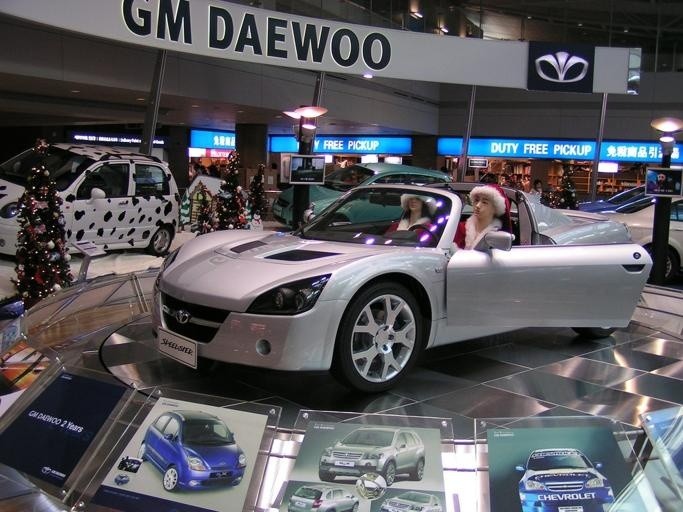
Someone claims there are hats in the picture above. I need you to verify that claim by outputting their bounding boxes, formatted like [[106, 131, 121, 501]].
[[400, 194, 437, 214], [470, 184, 516, 241]]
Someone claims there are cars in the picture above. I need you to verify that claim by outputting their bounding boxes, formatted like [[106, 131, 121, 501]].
[[514, 448, 615, 511], [0, 143, 182, 262], [573, 184, 682, 286], [380, 490, 442, 511], [270, 163, 452, 231], [136, 407, 247, 492]]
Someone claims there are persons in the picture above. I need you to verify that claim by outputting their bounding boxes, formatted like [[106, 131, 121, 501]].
[[453, 182, 516, 253], [656, 172, 666, 186], [499, 173, 531, 190], [529, 177, 544, 197], [386, 191, 437, 245]]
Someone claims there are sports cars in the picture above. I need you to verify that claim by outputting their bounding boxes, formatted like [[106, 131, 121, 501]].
[[154, 180, 652, 392]]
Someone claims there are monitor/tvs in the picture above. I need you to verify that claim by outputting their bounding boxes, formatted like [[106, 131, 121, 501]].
[[644, 167, 683, 197], [280, 153, 326, 186]]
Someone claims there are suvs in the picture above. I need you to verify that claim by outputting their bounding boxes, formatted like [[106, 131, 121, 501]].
[[317, 426, 427, 484], [287, 484, 362, 511]]
